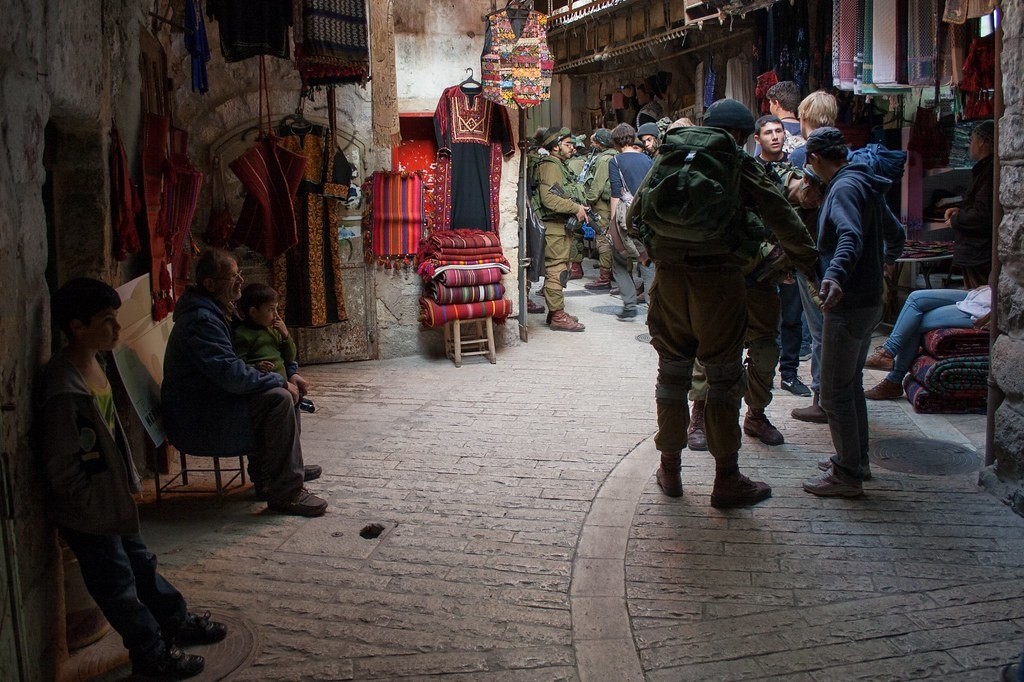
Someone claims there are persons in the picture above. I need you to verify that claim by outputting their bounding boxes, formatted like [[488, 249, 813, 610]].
[[36, 277, 227, 682], [526, 79, 995, 506], [161, 246, 327, 516]]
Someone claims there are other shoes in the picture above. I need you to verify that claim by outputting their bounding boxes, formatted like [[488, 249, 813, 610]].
[[268, 486, 328, 517], [546, 309, 586, 331], [527, 295, 545, 313], [256, 487, 269, 500]]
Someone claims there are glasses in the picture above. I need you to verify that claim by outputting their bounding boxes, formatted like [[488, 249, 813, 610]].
[[214, 270, 242, 283]]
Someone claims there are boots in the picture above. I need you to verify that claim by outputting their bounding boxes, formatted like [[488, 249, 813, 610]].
[[792, 392, 828, 423], [743, 408, 783, 446], [657, 455, 682, 498], [711, 463, 772, 508], [569, 263, 645, 318], [688, 401, 708, 451]]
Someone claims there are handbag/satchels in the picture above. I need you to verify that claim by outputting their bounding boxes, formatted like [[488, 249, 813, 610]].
[[609, 198, 650, 262]]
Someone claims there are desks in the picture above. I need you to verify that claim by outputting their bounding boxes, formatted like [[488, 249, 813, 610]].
[[868, 254, 969, 332]]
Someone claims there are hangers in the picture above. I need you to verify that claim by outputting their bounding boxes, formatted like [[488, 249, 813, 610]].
[[459, 68, 482, 95], [290, 106, 312, 127], [708, 39, 714, 59], [486, 0, 531, 20]]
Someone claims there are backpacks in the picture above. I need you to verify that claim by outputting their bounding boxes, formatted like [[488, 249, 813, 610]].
[[643, 126, 749, 260]]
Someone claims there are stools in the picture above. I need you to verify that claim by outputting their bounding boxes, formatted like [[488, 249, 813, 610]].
[[447, 315, 497, 367], [152, 437, 245, 503]]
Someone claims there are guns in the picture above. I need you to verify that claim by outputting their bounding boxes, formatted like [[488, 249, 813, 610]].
[[576, 146, 597, 184], [548, 181, 629, 262]]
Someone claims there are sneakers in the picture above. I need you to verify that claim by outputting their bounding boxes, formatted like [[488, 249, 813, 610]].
[[160, 610, 228, 644], [131, 637, 205, 678], [305, 464, 321, 482], [779, 348, 812, 360], [802, 456, 872, 498], [863, 379, 904, 401], [781, 377, 811, 396], [864, 348, 895, 371]]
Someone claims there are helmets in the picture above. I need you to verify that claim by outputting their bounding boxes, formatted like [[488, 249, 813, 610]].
[[705, 99, 757, 134], [542, 122, 659, 150], [800, 164, 827, 193]]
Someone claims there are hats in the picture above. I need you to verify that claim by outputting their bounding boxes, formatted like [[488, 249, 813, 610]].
[[975, 119, 994, 144], [803, 128, 845, 168], [656, 117, 694, 132]]
